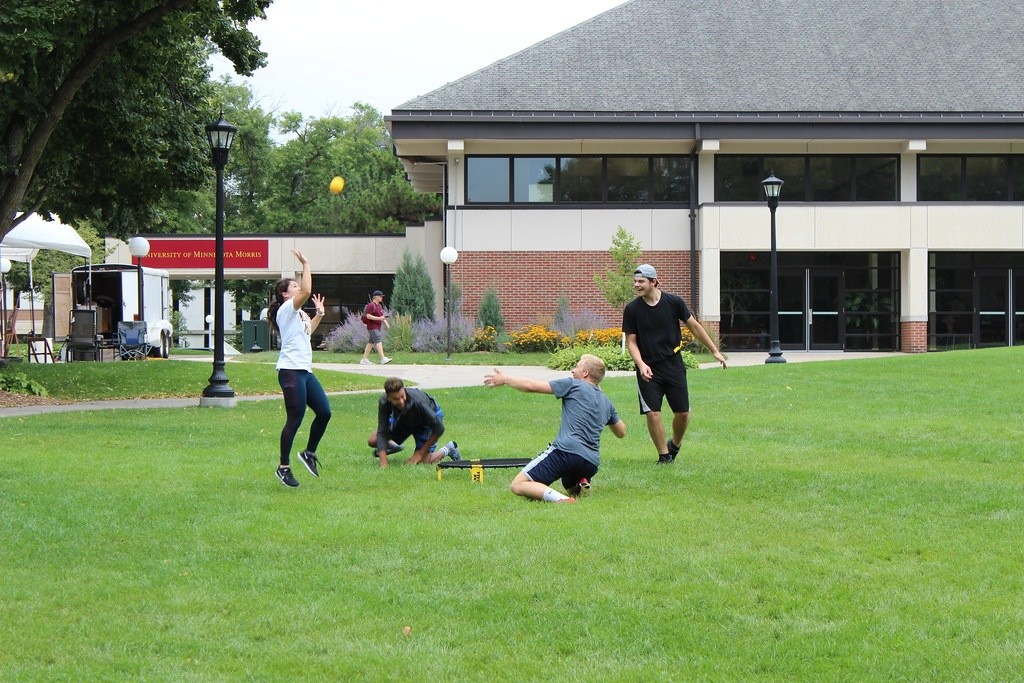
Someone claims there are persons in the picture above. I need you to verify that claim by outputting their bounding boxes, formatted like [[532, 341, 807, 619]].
[[485, 354, 626, 503], [266, 250, 331, 488], [622, 264, 726, 465], [368, 377, 461, 471], [360, 291, 393, 365]]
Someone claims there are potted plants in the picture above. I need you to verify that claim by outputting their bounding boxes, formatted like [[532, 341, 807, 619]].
[[832, 292, 893, 349], [721, 269, 770, 348]]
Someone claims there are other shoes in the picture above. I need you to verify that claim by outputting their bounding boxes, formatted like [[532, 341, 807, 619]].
[[557, 495, 575, 504], [656, 454, 674, 465], [447, 440, 462, 461], [576, 478, 590, 491], [374, 445, 405, 456], [667, 439, 680, 459]]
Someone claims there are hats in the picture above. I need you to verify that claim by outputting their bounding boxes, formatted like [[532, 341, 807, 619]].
[[373, 291, 385, 298], [634, 264, 660, 285]]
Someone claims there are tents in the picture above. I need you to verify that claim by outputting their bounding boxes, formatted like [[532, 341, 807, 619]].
[[0, 212, 91, 335]]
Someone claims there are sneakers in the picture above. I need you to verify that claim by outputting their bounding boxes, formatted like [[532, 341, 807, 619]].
[[360, 358, 374, 365], [275, 464, 300, 487], [296, 449, 323, 478], [381, 356, 392, 365]]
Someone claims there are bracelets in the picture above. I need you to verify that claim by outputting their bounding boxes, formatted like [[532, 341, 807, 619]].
[[316, 311, 324, 316]]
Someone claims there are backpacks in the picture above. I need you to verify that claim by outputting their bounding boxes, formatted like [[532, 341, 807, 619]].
[[361, 301, 377, 323]]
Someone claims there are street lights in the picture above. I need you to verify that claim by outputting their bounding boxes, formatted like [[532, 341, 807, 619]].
[[202, 103, 237, 397], [760, 171, 787, 364], [128, 237, 150, 360], [439, 246, 458, 361], [0, 258, 12, 358]]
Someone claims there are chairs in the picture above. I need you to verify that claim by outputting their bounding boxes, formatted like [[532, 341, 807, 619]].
[[65, 310, 101, 362], [113, 322, 153, 361]]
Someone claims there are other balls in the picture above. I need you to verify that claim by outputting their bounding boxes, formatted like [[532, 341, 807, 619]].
[[330, 175, 345, 194]]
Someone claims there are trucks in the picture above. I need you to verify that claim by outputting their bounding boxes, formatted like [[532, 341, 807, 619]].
[[53, 262, 173, 362]]
